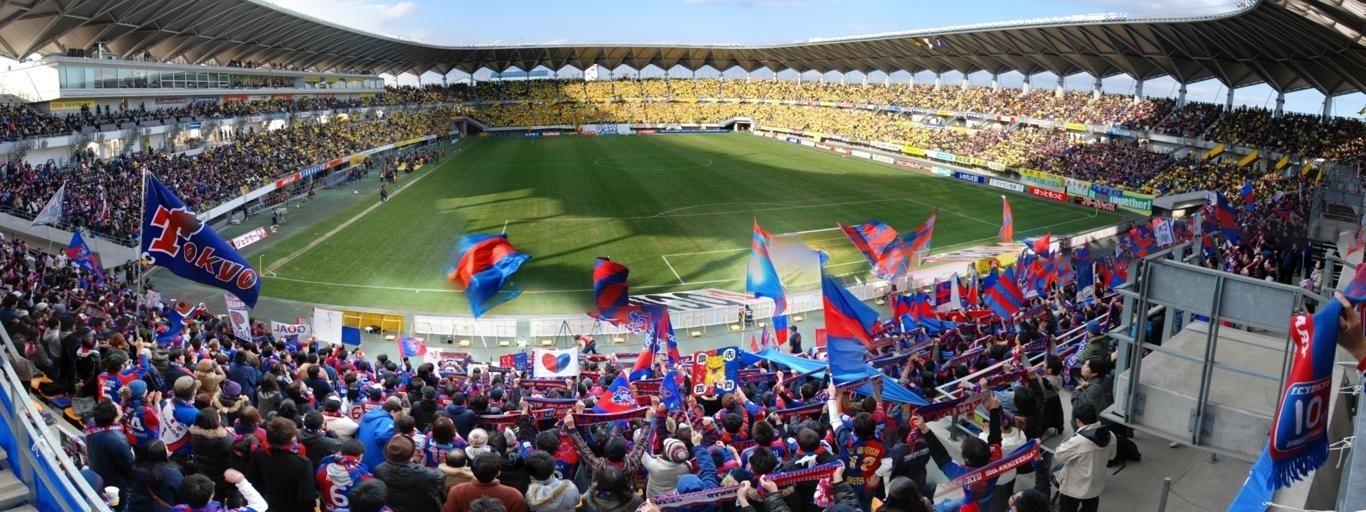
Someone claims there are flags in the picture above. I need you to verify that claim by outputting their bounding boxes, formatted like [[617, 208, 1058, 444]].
[[444, 212, 831, 367]]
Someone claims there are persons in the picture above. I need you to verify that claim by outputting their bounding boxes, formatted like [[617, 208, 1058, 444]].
[[447, 74, 835, 134], [446, 326, 835, 512], [0, 46, 446, 247], [837, 84, 1366, 511], [0, 234, 446, 512]]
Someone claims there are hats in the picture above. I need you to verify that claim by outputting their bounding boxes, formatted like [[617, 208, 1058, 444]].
[[342, 439, 366, 455], [173, 375, 202, 396], [663, 438, 689, 462], [420, 386, 436, 397], [128, 380, 148, 398], [677, 474, 704, 493], [222, 379, 242, 395], [1087, 320, 1101, 334], [382, 434, 416, 462], [468, 427, 489, 447]]
